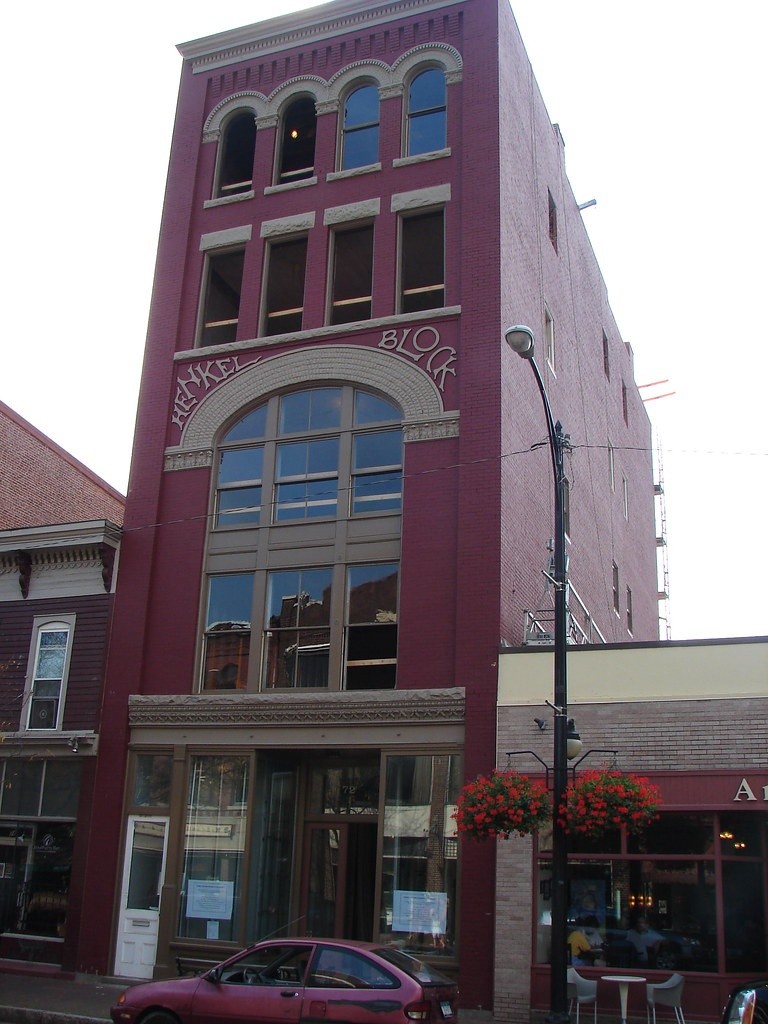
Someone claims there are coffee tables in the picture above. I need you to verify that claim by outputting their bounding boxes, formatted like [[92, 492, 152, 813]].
[[601, 975, 646, 1024]]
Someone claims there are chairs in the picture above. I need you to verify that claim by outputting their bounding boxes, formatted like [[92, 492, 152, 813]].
[[647, 973, 685, 1024], [567, 968, 598, 1024]]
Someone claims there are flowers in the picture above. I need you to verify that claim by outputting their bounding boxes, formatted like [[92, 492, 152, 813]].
[[557, 760, 665, 841], [449, 768, 552, 844]]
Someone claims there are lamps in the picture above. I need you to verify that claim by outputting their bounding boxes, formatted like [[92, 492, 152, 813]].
[[534, 717, 549, 731], [66, 733, 79, 753]]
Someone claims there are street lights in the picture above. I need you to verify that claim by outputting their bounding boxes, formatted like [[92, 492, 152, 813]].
[[504, 324, 583, 1024]]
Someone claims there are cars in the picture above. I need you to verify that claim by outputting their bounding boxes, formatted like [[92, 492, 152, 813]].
[[110, 937, 461, 1024]]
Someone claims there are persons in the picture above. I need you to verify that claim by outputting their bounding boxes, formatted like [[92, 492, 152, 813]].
[[566, 910, 713, 970]]
